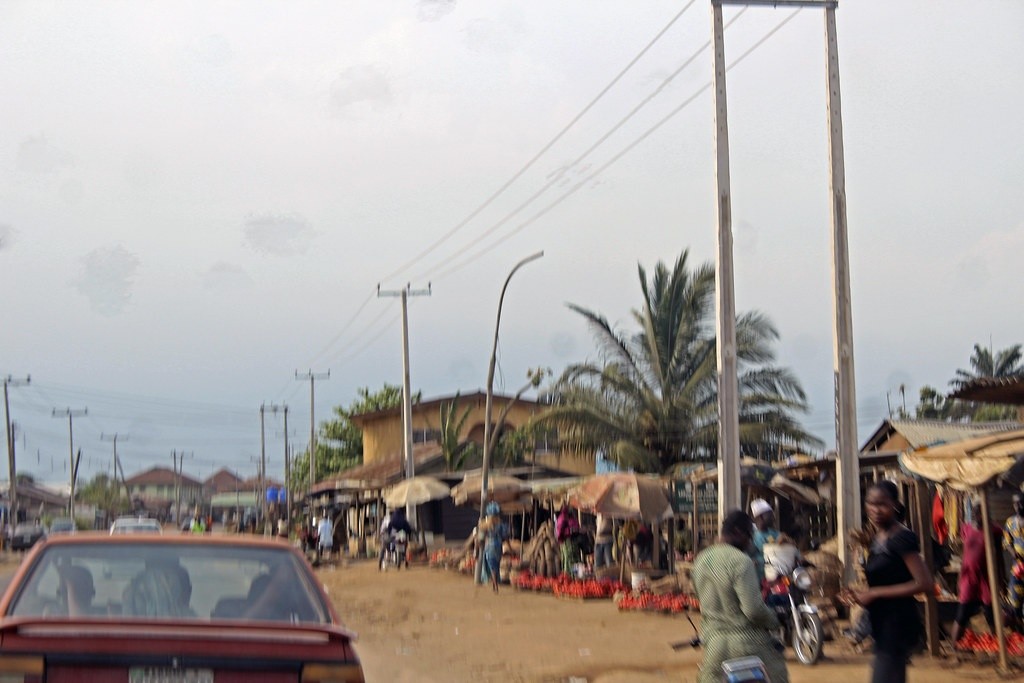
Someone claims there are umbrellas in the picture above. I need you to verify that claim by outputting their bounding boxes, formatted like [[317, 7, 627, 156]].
[[567, 472, 675, 588], [455, 471, 531, 506], [383, 476, 451, 531]]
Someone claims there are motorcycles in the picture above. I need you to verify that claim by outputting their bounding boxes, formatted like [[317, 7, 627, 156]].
[[381, 530, 417, 572], [757, 557, 824, 666]]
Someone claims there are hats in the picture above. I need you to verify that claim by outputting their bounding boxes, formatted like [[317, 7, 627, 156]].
[[485, 501, 499, 516], [751, 499, 777, 522]]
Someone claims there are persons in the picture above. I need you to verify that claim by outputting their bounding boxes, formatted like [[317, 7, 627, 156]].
[[742, 498, 799, 607], [591, 512, 652, 570], [272, 507, 335, 558], [181, 513, 213, 535], [838, 479, 932, 683], [33, 552, 298, 622], [453, 501, 513, 591], [378, 510, 413, 571], [689, 509, 791, 683], [944, 491, 1024, 668]]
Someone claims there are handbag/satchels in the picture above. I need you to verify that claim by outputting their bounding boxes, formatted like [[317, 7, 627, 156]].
[[405, 542, 413, 562]]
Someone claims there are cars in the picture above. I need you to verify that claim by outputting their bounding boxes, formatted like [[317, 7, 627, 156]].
[[49, 518, 78, 533], [108, 520, 164, 535], [10, 520, 48, 553], [0, 534, 367, 683]]
